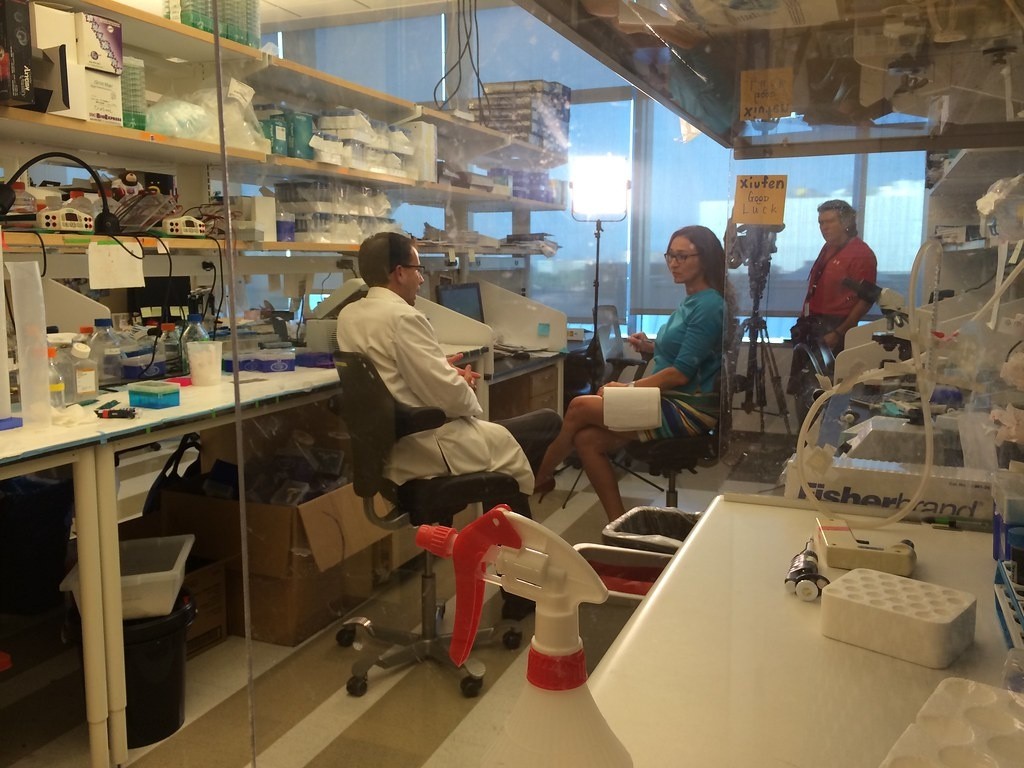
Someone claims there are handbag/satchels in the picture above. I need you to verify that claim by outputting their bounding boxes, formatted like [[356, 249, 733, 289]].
[[144, 432, 203, 515]]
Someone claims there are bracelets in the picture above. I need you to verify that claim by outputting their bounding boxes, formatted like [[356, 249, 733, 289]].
[[628, 381, 635, 387]]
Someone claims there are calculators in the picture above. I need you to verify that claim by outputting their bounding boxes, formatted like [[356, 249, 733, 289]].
[[850, 388, 921, 411]]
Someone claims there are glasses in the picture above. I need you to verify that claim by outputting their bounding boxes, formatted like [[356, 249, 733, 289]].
[[664, 251, 703, 265], [389, 264, 426, 277]]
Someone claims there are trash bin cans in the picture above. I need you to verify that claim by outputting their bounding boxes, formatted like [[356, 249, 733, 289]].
[[599, 505, 705, 554], [60, 583, 198, 751], [571, 542, 675, 677]]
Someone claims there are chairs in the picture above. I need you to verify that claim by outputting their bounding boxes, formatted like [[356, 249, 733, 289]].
[[335, 353, 521, 696], [591, 304, 737, 505]]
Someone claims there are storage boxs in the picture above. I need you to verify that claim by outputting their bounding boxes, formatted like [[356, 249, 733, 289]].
[[470, 78, 571, 205], [58, 453, 427, 662], [128, 380, 180, 409], [0, 0, 123, 124], [223, 348, 296, 372]]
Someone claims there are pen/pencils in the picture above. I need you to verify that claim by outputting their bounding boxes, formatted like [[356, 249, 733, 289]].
[[922, 516, 992, 524], [949, 520, 993, 532]]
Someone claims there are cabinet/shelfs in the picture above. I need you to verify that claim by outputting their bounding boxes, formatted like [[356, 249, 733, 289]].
[[413, 491, 1024, 768], [0, 0, 568, 766]]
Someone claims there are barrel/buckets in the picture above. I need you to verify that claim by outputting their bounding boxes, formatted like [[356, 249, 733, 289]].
[[72, 589, 196, 748]]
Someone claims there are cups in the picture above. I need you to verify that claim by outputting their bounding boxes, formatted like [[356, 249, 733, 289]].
[[186, 340, 224, 386]]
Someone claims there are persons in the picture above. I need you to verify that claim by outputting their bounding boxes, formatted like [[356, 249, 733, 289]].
[[334, 234, 566, 620], [533, 227, 729, 522], [788, 201, 880, 430]]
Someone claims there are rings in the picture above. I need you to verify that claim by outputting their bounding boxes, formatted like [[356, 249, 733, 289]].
[[602, 385, 607, 388]]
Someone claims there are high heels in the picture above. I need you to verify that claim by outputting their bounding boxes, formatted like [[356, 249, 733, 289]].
[[532, 477, 555, 504]]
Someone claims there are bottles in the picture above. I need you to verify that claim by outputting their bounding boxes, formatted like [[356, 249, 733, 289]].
[[161, 323, 184, 376], [180, 313, 211, 374], [48, 317, 123, 411], [0, 181, 121, 232]]
